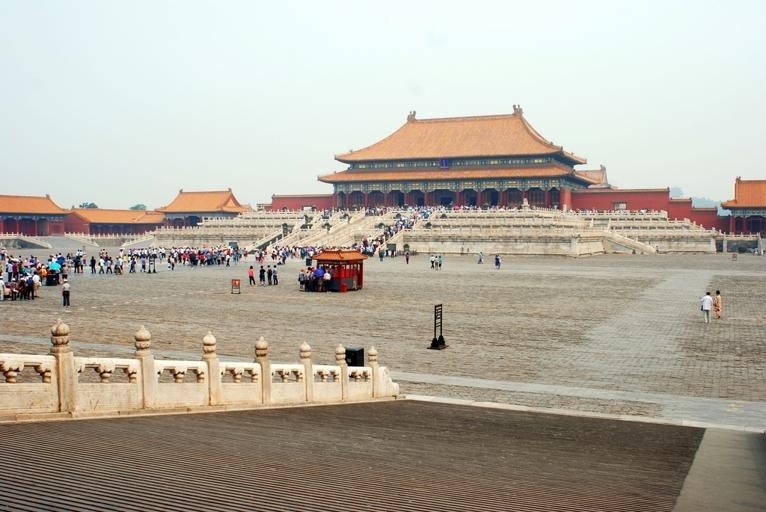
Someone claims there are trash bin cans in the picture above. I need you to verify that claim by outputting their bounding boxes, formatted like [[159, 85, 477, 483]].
[[345, 346, 364, 366]]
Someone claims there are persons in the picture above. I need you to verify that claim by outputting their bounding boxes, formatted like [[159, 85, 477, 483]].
[[0, 247, 87, 306], [495, 254, 502, 268], [352, 214, 430, 247], [700, 291, 715, 323], [249, 248, 336, 292], [325, 204, 668, 215], [431, 255, 442, 270], [91, 247, 247, 274], [356, 247, 409, 263], [713, 289, 722, 318], [477, 251, 485, 264]]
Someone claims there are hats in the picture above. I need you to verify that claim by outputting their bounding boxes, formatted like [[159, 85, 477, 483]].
[[0, 276, 32, 282]]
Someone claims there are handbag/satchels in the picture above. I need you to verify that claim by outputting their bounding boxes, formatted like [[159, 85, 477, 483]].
[[700, 304, 703, 311]]
